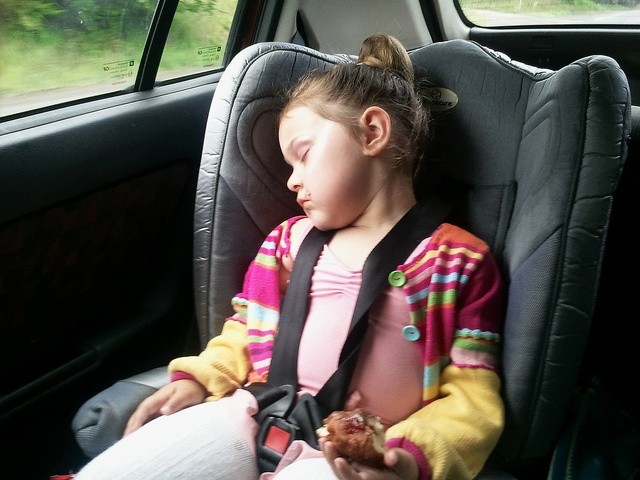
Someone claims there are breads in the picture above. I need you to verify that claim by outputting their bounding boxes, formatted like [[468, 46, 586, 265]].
[[318, 408, 389, 467]]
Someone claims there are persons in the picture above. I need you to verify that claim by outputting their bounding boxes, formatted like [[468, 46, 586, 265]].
[[122, 33, 504, 480]]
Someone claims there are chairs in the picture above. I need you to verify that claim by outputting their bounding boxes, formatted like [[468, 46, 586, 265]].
[[73, 38, 630, 474]]
